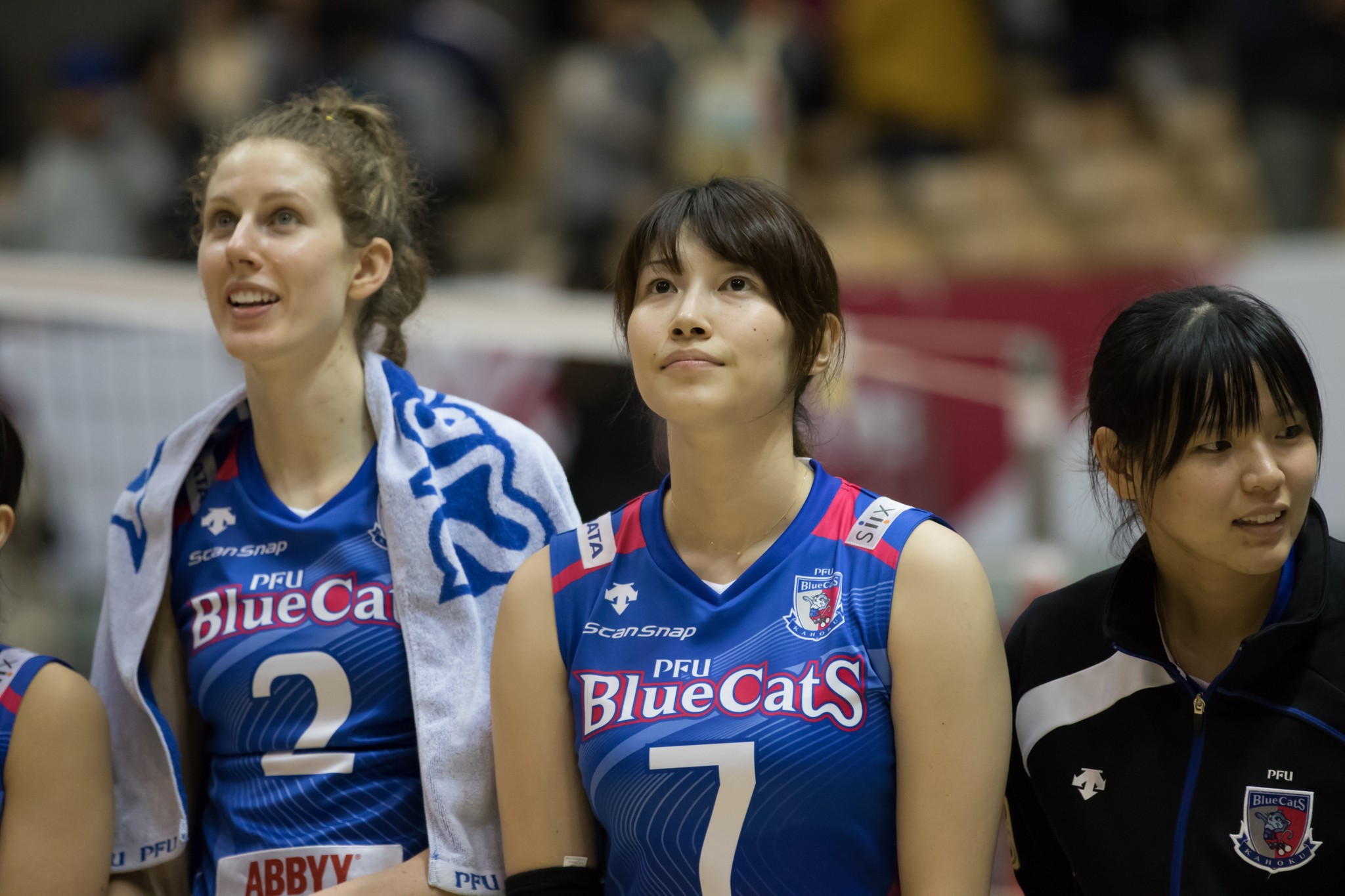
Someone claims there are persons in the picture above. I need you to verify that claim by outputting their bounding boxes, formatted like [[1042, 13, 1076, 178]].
[[0, 409, 115, 896], [489, 180, 1011, 896], [993, 284, 1345, 896], [86, 81, 583, 896], [0, 0, 1345, 299]]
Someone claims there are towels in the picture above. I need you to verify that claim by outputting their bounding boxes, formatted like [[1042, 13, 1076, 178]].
[[78, 346, 590, 896]]
